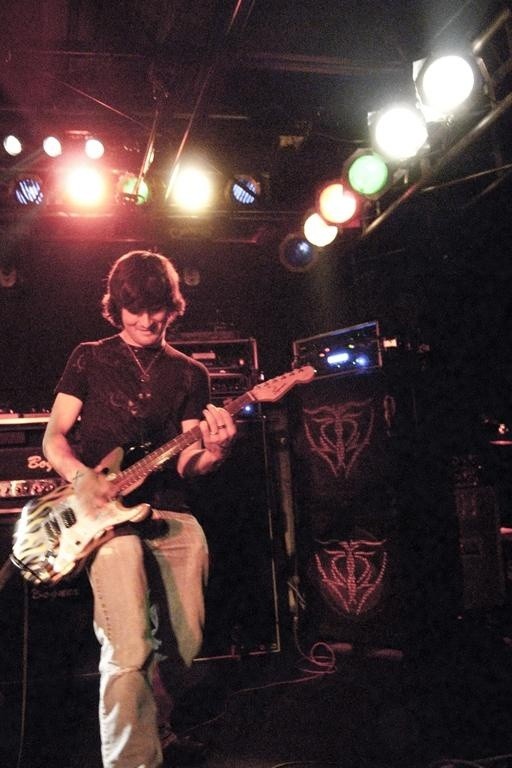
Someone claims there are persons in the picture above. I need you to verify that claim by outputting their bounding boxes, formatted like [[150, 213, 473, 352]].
[[41, 249, 243, 768]]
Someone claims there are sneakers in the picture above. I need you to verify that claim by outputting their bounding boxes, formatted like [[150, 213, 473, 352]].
[[162, 733, 209, 764]]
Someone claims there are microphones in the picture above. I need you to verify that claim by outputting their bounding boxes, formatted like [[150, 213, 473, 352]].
[[331, 640, 406, 666]]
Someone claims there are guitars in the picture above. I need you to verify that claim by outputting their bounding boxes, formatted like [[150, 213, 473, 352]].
[[11, 364, 315, 586]]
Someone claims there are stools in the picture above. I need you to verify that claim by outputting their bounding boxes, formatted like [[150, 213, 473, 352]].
[[13, 569, 156, 768]]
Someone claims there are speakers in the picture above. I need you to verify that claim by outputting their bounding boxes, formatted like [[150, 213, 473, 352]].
[[285, 366, 416, 654], [1, 443, 101, 679], [163, 418, 283, 663]]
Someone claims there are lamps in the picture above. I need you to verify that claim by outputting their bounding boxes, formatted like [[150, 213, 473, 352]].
[[276, 27, 476, 274]]
